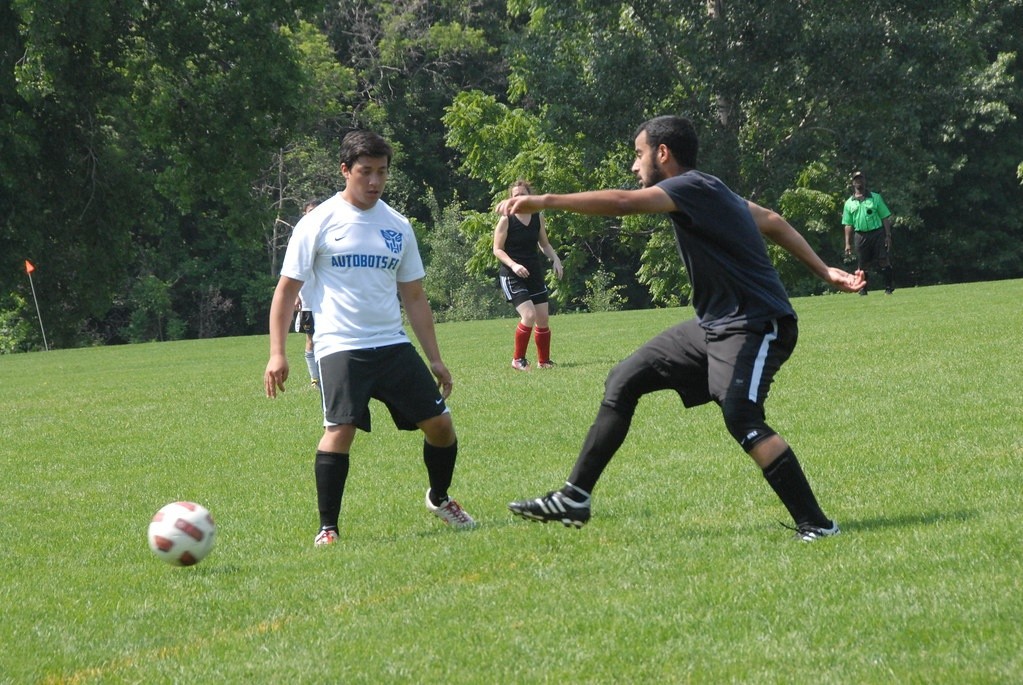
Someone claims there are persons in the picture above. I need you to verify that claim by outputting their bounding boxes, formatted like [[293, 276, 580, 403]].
[[264, 133, 479, 546], [495, 118, 866, 543], [294, 197, 323, 389], [843, 170, 895, 295], [492, 180, 563, 372]]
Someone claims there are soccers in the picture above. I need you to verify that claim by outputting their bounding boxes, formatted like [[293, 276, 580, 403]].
[[147, 499, 216, 568]]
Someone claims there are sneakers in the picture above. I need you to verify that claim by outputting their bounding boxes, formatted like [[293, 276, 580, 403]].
[[314, 531, 341, 548], [508, 490, 592, 529], [793, 520, 840, 544], [537, 361, 556, 367], [424, 487, 476, 528], [511, 357, 531, 370]]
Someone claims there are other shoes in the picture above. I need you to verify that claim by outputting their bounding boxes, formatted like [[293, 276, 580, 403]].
[[859, 288, 866, 294], [885, 286, 895, 295]]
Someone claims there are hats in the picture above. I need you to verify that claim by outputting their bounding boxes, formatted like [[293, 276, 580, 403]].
[[851, 172, 866, 178]]
